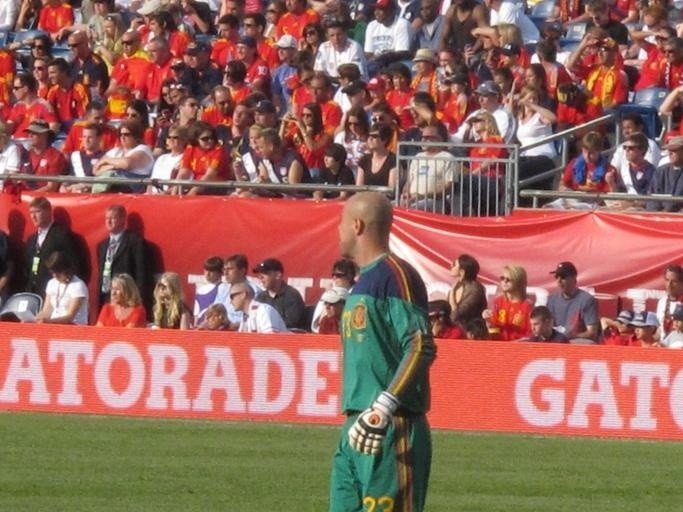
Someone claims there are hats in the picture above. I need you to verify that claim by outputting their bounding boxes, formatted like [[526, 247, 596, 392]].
[[252, 258, 284, 273], [496, 42, 521, 55], [662, 136, 683, 151], [444, 72, 468, 85], [474, 80, 501, 96], [549, 262, 577, 276], [614, 304, 683, 328], [320, 287, 349, 304]]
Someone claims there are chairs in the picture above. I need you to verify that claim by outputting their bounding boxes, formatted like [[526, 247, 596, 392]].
[[0, 2, 682, 218], [1, 291, 603, 347]]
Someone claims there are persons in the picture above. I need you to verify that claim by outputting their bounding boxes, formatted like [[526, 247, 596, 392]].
[[310, 260, 357, 334], [0, 196, 89, 324], [95, 203, 311, 334], [329, 191, 435, 512], [428, 254, 683, 349], [0, 2, 683, 217]]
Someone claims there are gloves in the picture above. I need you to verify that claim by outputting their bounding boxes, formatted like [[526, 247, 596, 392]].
[[348, 391, 401, 456]]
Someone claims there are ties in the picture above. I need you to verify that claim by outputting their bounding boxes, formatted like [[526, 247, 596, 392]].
[[101, 239, 115, 295]]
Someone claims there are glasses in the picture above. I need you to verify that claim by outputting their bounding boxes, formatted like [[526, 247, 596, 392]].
[[501, 276, 510, 282], [332, 273, 344, 278], [13, 0, 442, 141], [597, 47, 608, 51], [230, 292, 239, 299], [622, 144, 636, 150], [663, 49, 672, 53]]
